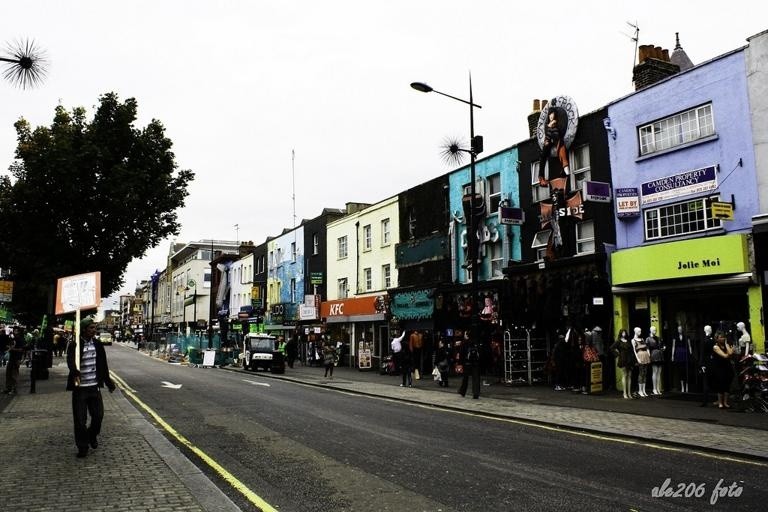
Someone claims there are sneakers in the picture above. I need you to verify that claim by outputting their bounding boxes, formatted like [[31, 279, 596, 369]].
[[458, 390, 479, 399], [75, 433, 98, 459], [399, 384, 413, 389], [2, 388, 17, 395], [554, 385, 589, 395]]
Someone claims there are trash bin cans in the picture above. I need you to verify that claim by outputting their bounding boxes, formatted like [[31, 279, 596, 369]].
[[32, 349, 49, 380]]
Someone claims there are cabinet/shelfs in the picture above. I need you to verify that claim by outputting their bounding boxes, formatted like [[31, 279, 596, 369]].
[[501, 327, 548, 388]]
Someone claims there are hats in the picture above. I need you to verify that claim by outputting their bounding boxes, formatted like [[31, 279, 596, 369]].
[[80, 316, 96, 330]]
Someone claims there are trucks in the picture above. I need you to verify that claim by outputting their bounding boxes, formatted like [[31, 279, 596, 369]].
[[152, 327, 170, 341]]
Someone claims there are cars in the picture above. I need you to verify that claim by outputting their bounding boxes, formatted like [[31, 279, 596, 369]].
[[94, 334, 100, 340], [99, 331, 113, 346]]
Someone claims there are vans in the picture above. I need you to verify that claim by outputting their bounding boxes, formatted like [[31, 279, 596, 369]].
[[134, 328, 144, 338], [113, 331, 122, 338]]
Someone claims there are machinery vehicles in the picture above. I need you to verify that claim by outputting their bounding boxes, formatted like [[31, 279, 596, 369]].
[[242, 332, 286, 375]]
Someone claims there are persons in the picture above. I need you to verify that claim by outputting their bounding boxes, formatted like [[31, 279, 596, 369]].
[[0, 316, 147, 459], [434, 338, 448, 386], [400, 340, 415, 389], [456, 328, 481, 398], [322, 339, 337, 379], [376, 296, 387, 314], [712, 330, 735, 409], [548, 325, 574, 390], [275, 336, 298, 368], [736, 321, 751, 358], [581, 325, 720, 409], [477, 295, 495, 321], [409, 331, 424, 380], [390, 329, 406, 373]]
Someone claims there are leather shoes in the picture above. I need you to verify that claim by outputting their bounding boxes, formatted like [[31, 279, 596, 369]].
[[622, 391, 663, 400]]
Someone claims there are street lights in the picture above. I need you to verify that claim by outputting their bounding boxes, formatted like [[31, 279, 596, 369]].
[[177, 284, 186, 328], [407, 68, 489, 398], [127, 292, 133, 328], [112, 299, 118, 327], [185, 278, 197, 334], [146, 282, 150, 323]]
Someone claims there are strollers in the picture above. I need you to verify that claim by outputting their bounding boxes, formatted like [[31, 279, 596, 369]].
[[379, 354, 396, 374]]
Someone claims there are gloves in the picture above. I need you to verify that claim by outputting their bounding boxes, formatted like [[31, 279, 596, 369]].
[[107, 382, 117, 393], [70, 367, 81, 378]]
